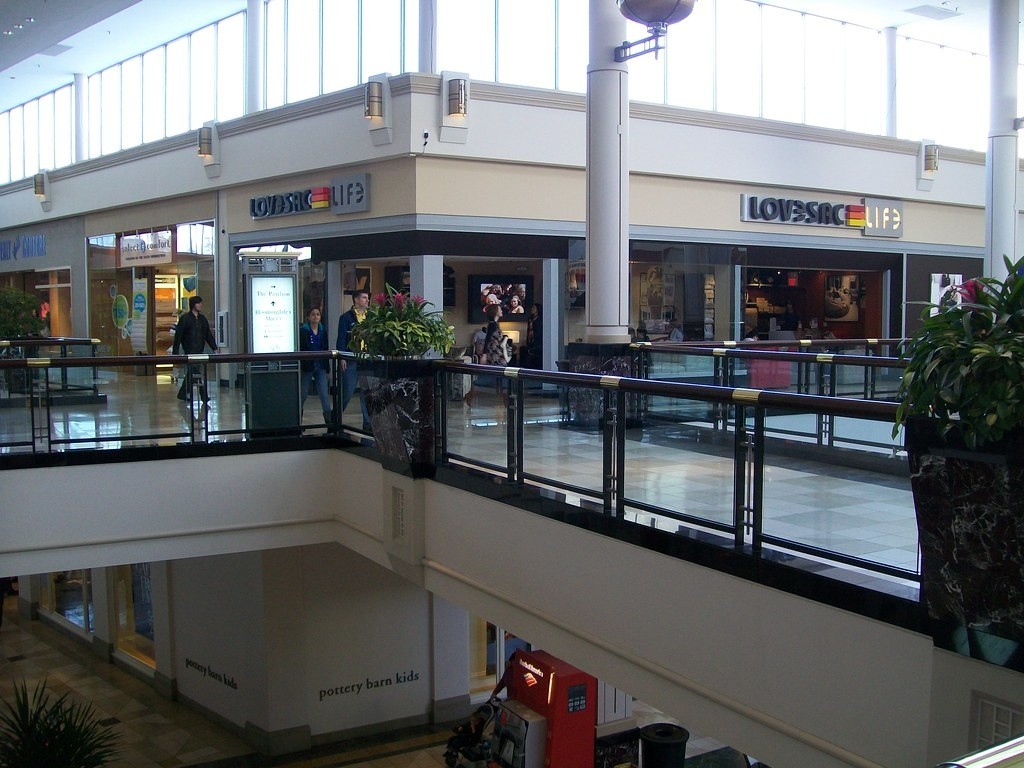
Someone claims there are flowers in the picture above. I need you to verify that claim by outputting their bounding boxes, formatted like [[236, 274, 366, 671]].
[[893, 252, 1024, 452], [347, 285, 456, 360]]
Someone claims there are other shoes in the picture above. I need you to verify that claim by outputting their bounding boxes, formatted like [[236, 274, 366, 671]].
[[362, 422, 372, 432], [177, 394, 190, 401], [200, 396, 210, 402]]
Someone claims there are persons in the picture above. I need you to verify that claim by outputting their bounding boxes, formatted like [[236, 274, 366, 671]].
[[526, 302, 542, 351], [742, 324, 758, 342], [485, 285, 524, 314], [172, 296, 218, 402], [464, 304, 512, 406], [629, 320, 684, 342], [300, 307, 332, 433], [337, 290, 372, 443]]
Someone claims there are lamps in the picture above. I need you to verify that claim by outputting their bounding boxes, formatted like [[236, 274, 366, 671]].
[[364, 82, 382, 119], [197, 127, 212, 156], [925, 145, 939, 171], [449, 79, 468, 115]]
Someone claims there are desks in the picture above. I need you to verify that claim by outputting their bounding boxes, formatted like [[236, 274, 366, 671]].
[[746, 347, 798, 388]]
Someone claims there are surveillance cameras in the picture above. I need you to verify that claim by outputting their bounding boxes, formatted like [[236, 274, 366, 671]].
[[422, 130, 431, 145]]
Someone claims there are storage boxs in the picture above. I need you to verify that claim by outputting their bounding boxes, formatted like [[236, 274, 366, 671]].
[[757, 298, 786, 312]]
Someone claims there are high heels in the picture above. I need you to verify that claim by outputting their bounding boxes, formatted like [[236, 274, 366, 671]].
[[503, 394, 510, 408], [464, 392, 473, 407]]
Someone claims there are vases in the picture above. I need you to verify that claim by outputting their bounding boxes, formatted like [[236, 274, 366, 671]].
[[379, 337, 430, 356], [902, 413, 1024, 625]]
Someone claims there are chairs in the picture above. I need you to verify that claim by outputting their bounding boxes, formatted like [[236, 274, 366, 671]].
[[838, 349, 872, 385]]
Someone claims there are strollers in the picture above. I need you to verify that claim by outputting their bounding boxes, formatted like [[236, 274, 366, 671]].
[[445, 704, 493, 768]]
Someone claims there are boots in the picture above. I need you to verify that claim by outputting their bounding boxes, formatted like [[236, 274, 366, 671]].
[[321, 410, 338, 435]]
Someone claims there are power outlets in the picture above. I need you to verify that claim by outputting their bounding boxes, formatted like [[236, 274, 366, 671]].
[[33, 174, 44, 195]]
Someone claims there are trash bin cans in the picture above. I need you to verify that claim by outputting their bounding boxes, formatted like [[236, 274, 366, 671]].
[[639, 723, 691, 768], [555, 359, 569, 415]]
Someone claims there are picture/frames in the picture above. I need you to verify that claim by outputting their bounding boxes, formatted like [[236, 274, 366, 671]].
[[343, 266, 372, 295], [640, 273, 676, 323], [824, 274, 860, 322]]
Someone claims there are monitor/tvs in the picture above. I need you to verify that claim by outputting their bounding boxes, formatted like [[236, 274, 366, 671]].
[[468, 273, 534, 323]]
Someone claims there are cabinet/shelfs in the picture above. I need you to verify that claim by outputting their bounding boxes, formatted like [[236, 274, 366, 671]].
[[748, 283, 772, 306]]
